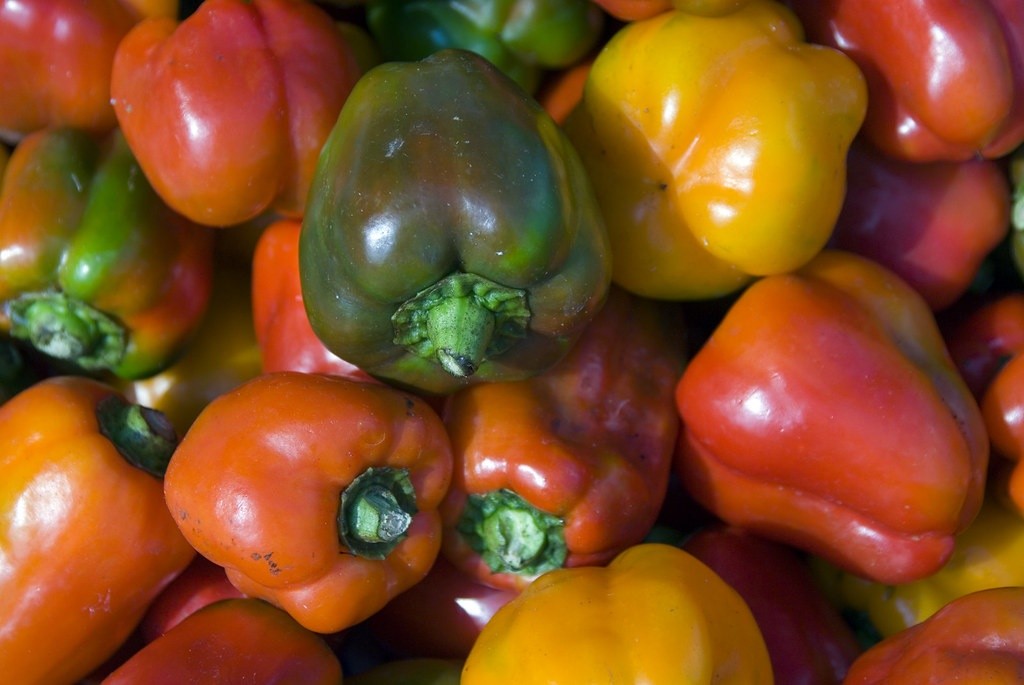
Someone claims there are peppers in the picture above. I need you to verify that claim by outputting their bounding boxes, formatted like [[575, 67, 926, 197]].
[[0, 0, 1024, 685]]
